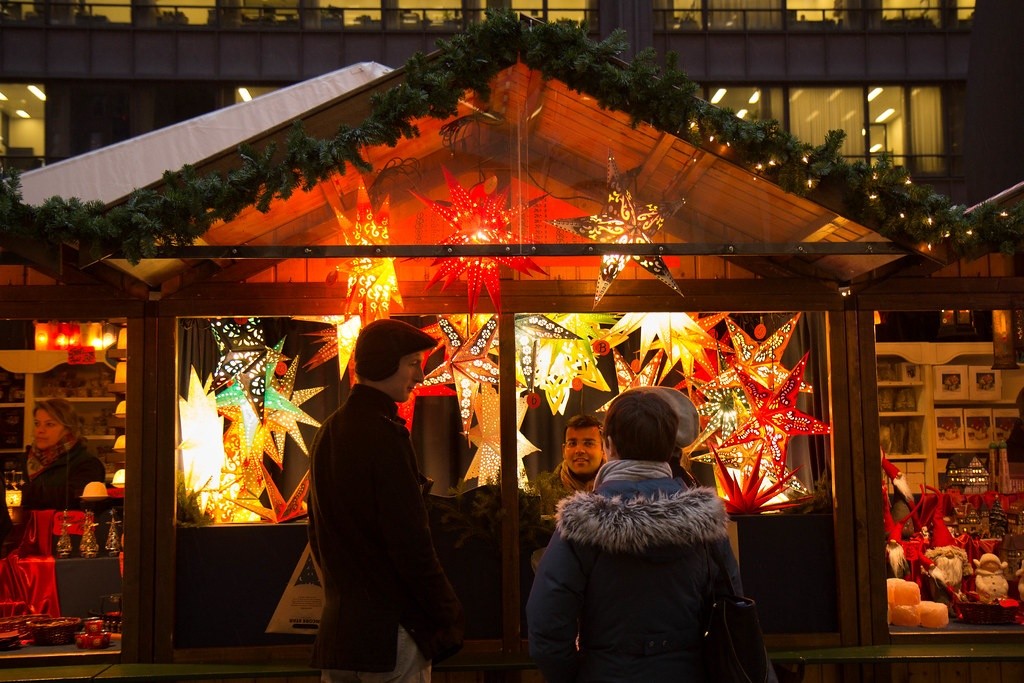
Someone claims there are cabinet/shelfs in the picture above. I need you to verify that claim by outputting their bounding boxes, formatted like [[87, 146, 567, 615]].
[[0, 349, 127, 510], [876, 342, 1024, 499]]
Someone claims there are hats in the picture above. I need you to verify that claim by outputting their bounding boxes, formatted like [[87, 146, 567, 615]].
[[353, 319, 438, 363], [633, 385, 700, 450]]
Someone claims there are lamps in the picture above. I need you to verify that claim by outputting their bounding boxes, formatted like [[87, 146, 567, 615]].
[[5, 323, 129, 507], [990, 309, 1020, 370]]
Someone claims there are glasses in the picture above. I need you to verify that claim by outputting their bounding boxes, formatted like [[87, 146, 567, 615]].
[[564, 441, 600, 449]]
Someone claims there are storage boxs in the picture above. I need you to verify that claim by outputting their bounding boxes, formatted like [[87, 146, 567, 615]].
[[876, 360, 1020, 494]]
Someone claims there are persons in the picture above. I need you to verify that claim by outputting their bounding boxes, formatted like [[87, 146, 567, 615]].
[[0, 454, 14, 541], [307, 319, 465, 683], [21, 399, 109, 510], [526, 385, 780, 683], [524, 413, 604, 536]]
